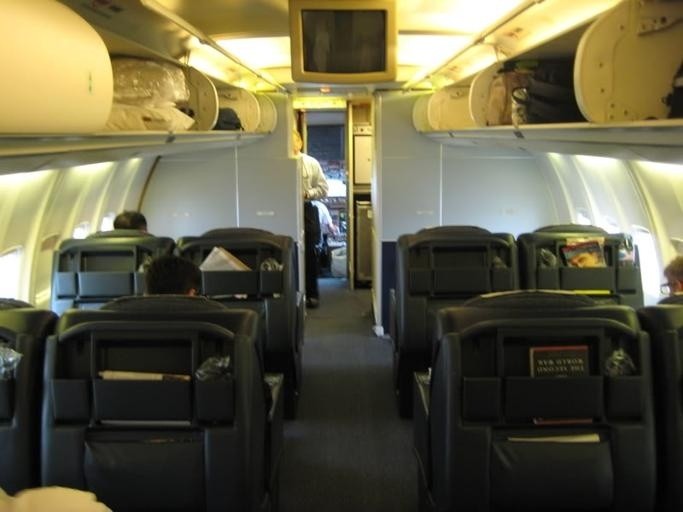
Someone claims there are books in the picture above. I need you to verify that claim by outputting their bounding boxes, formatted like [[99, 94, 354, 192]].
[[561, 239, 607, 269], [527, 342, 594, 425], [564, 235, 606, 255], [198, 246, 256, 299]]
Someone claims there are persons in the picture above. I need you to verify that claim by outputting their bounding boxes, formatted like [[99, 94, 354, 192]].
[[145, 254, 202, 297], [658, 256, 683, 295], [308, 197, 338, 278], [291, 131, 330, 308], [113, 211, 147, 232], [566, 246, 603, 268]]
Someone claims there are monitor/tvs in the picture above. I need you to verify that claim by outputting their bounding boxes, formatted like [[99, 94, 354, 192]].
[[289, 0, 396, 84]]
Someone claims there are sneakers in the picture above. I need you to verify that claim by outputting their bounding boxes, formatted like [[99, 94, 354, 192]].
[[307, 296, 319, 309]]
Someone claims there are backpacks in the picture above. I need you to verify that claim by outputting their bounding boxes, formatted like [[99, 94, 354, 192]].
[[512, 59, 586, 124]]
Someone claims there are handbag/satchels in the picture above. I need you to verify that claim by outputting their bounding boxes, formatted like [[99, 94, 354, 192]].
[[214, 107, 241, 130], [107, 59, 198, 132]]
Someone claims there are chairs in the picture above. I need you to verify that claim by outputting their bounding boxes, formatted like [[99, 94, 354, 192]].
[[379, 225, 683, 511], [0, 228, 306, 512]]
